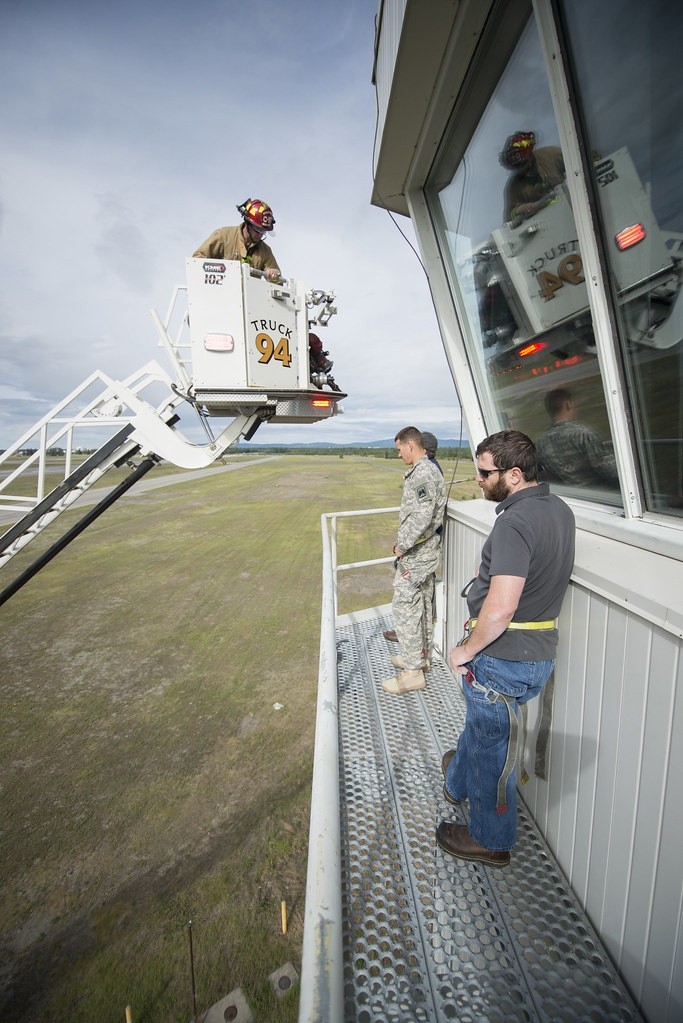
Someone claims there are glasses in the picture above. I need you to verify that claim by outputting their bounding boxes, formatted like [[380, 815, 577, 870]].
[[478, 466, 525, 478], [248, 223, 266, 235]]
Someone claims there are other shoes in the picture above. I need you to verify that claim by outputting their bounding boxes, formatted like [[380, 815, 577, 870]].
[[383, 630, 398, 642], [423, 648, 429, 657]]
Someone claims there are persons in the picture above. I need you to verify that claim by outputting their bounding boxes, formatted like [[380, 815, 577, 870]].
[[533, 387, 618, 491], [435, 429, 576, 868], [498, 130, 567, 230], [193, 199, 283, 287], [381, 426, 447, 694]]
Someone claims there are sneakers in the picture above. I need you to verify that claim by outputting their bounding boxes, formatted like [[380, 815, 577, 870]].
[[391, 655, 430, 671], [382, 668, 426, 694]]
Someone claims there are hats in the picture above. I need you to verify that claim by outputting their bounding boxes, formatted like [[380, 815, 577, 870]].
[[421, 432, 438, 452]]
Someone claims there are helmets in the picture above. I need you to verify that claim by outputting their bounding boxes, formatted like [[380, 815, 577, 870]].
[[236, 198, 276, 231], [498, 131, 535, 170]]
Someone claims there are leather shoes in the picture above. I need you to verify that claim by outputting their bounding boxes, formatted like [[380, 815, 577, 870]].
[[442, 750, 460, 804], [435, 822, 510, 867]]
